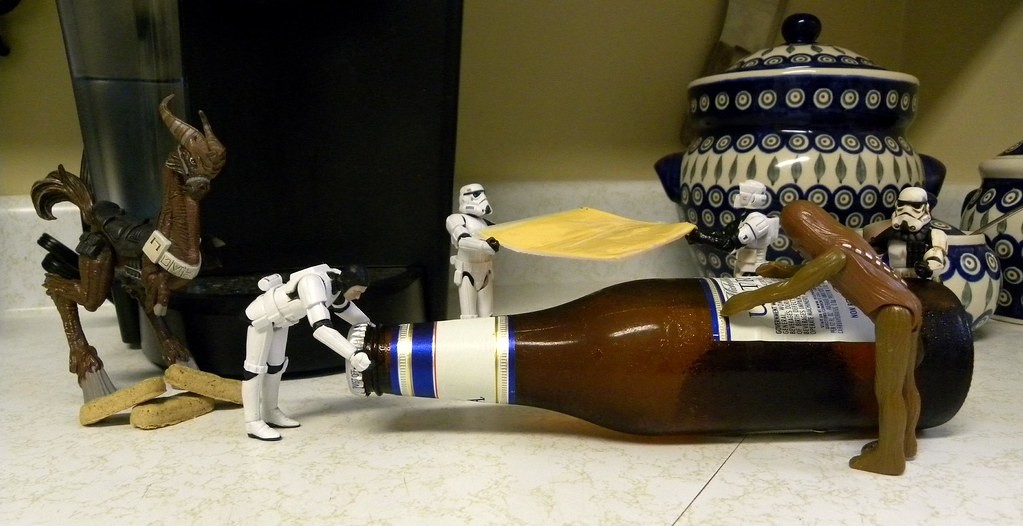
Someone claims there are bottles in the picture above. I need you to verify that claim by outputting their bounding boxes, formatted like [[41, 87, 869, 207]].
[[345, 277, 975, 437]]
